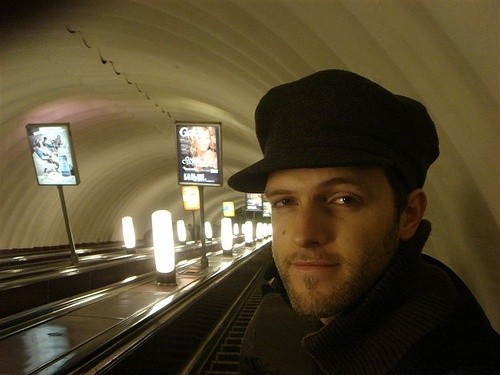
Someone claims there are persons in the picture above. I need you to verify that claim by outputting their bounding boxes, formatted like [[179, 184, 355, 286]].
[[186, 126, 218, 172], [226, 69, 499, 375]]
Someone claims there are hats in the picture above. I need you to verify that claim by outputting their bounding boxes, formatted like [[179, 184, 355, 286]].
[[225, 68, 441, 194]]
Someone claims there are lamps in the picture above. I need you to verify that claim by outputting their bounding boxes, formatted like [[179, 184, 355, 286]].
[[121, 216, 136, 253], [152, 210, 178, 286], [176, 220, 186, 246], [204, 217, 273, 257]]
